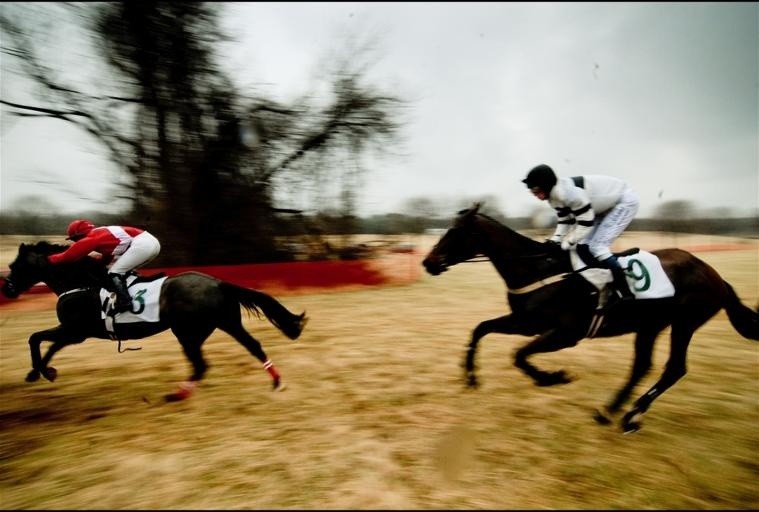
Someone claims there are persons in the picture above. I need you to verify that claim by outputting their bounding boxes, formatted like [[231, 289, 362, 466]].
[[47, 220, 161, 313], [521, 163, 640, 300]]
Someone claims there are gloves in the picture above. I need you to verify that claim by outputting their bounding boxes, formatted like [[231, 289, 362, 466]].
[[540, 244, 561, 259]]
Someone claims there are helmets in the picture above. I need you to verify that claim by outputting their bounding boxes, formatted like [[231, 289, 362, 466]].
[[65, 220, 93, 240], [522, 165, 557, 188]]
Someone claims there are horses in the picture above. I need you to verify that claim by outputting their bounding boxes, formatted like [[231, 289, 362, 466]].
[[1, 239, 310, 403], [421, 199, 758, 438]]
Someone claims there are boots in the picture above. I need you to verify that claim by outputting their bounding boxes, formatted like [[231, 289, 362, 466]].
[[107, 273, 133, 317], [600, 254, 635, 313]]
